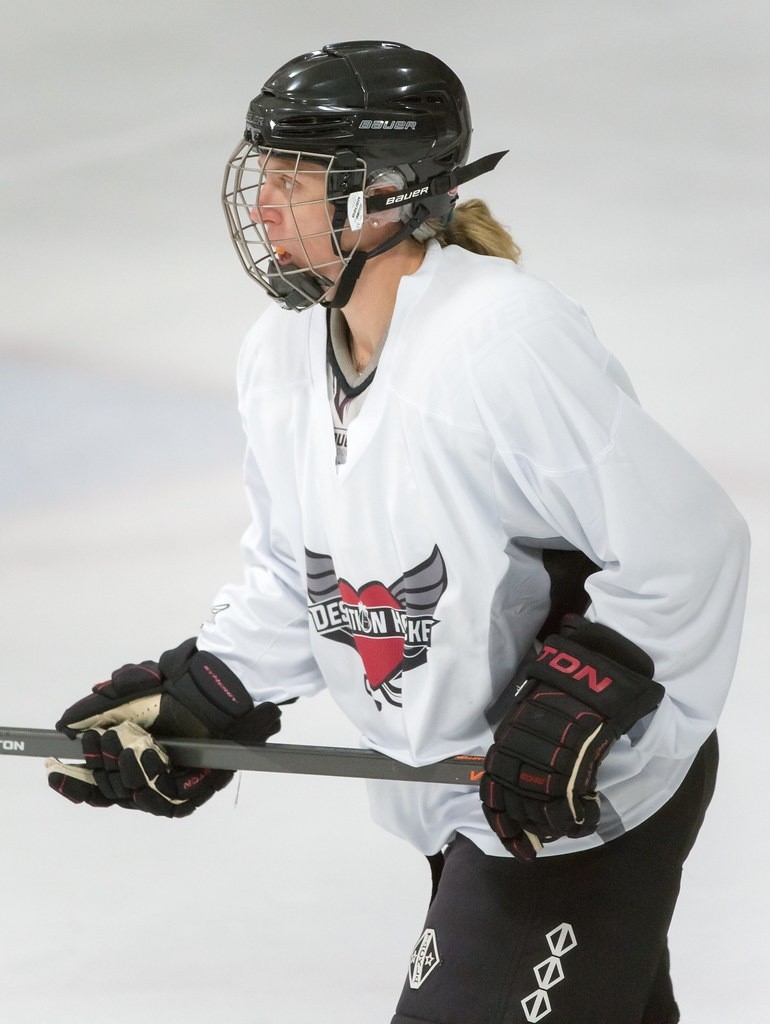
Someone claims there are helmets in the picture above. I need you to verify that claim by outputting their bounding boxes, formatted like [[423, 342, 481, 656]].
[[221, 40, 509, 314]]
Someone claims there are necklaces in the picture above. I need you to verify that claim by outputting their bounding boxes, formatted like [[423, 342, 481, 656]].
[[349, 344, 376, 381]]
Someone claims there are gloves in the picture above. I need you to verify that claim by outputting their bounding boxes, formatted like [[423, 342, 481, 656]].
[[45, 637, 281, 818], [480, 615, 665, 864]]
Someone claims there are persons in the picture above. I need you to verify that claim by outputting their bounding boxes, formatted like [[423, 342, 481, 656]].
[[46, 40, 751, 1024]]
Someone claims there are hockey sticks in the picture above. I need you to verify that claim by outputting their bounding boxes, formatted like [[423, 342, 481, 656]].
[[0, 725, 487, 786]]
